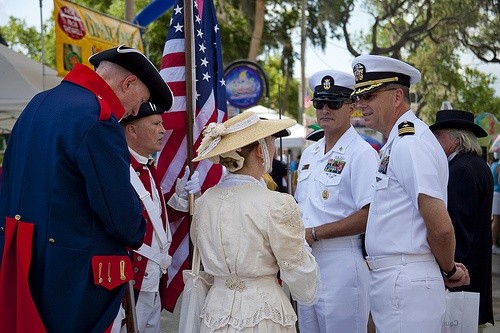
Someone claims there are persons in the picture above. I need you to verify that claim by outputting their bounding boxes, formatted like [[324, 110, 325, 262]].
[[0, 43, 175, 333], [266, 159, 289, 193], [292, 68, 380, 333], [105, 98, 173, 333], [490, 152, 500, 255], [429, 109, 494, 326], [349, 54, 471, 333], [276, 148, 302, 195], [186, 109, 320, 333]]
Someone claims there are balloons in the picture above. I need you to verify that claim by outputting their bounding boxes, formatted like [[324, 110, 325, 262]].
[[474, 112, 500, 147]]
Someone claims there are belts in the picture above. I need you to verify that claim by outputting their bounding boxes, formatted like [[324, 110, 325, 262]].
[[367, 254, 433, 269], [308, 238, 362, 248]]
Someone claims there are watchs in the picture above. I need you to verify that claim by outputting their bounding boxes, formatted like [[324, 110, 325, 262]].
[[442, 265, 457, 278]]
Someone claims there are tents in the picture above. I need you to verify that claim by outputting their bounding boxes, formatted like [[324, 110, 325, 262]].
[[242, 105, 306, 150]]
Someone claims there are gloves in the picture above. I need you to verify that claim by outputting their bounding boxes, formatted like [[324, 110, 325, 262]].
[[176, 165, 200, 199]]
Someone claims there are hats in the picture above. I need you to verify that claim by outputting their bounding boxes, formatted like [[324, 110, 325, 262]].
[[350, 54, 422, 96], [305, 128, 326, 142], [429, 109, 487, 138], [88, 44, 174, 124], [308, 68, 359, 103], [191, 111, 297, 163], [119, 100, 163, 126], [259, 114, 291, 138]]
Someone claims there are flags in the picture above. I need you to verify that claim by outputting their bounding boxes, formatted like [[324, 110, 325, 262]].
[[156, 0, 229, 312]]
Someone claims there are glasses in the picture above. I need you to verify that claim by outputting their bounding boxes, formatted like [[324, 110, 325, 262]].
[[355, 88, 397, 101], [312, 99, 352, 110]]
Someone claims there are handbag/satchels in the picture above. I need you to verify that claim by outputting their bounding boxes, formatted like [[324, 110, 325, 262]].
[[442, 279, 480, 333], [179, 243, 213, 333]]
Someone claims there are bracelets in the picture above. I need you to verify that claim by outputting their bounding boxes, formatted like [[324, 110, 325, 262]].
[[312, 227, 317, 242]]
